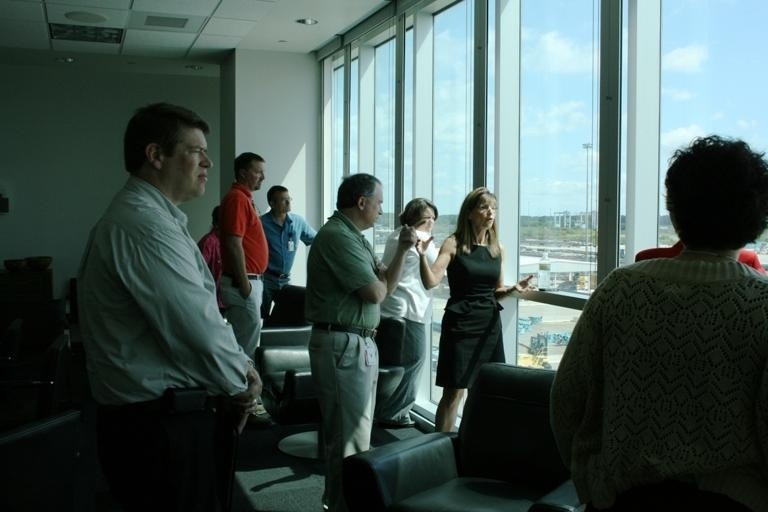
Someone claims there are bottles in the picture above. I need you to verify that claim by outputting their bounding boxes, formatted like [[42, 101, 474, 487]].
[[537, 253, 552, 291]]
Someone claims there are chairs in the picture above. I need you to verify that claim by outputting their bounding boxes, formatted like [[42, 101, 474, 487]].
[[340, 362, 588, 512], [259, 284, 313, 347], [255, 316, 407, 424]]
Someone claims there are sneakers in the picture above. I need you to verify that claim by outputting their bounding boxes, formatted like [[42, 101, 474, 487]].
[[388, 415, 419, 430]]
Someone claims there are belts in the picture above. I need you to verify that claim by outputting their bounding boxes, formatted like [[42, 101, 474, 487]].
[[266, 270, 292, 279], [221, 270, 265, 281], [311, 321, 379, 342], [86, 385, 248, 428]]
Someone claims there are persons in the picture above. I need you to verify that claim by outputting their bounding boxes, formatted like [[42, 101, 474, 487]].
[[73, 102, 264, 512], [375, 198, 448, 429], [218, 151, 270, 417], [195, 206, 228, 319], [303, 172, 420, 512], [547, 135, 767, 512], [635, 239, 765, 274], [258, 184, 318, 326], [414, 184, 538, 435]]
